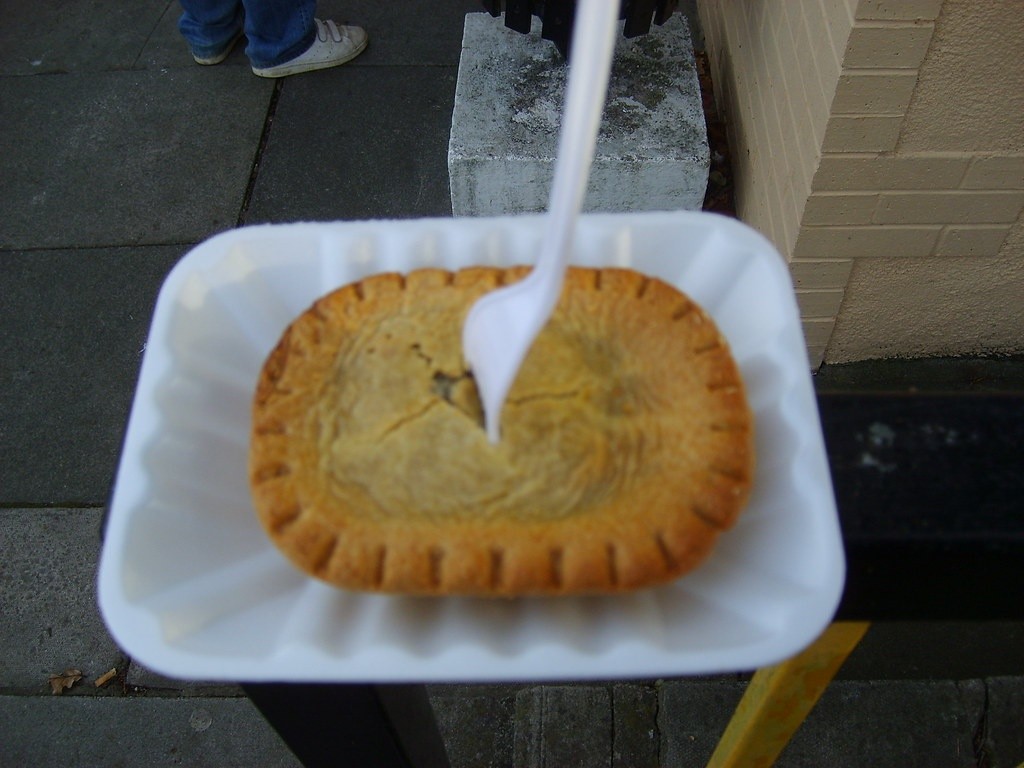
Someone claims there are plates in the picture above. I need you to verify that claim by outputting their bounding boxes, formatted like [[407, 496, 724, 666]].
[[94, 211, 849, 682]]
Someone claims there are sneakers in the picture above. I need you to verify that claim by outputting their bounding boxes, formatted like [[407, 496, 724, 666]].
[[193, 31, 242, 65], [252, 18, 368, 77]]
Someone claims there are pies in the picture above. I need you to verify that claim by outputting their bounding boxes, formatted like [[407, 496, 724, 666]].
[[248, 265, 757, 598]]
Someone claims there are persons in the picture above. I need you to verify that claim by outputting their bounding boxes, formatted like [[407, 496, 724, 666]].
[[177, 0, 369, 78]]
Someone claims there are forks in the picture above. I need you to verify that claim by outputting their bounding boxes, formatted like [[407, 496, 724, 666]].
[[460, 0, 620, 443]]
